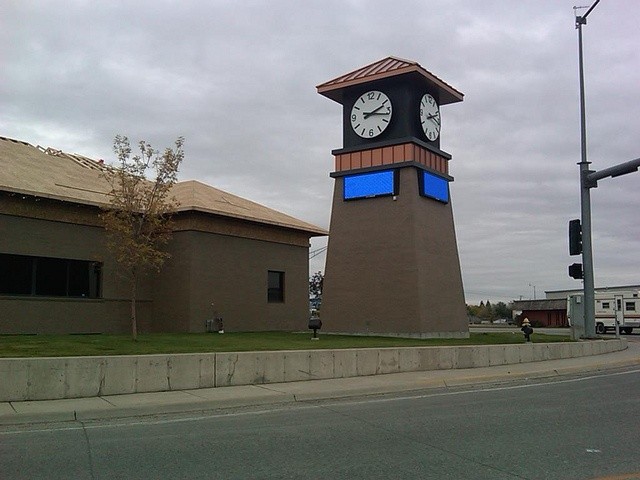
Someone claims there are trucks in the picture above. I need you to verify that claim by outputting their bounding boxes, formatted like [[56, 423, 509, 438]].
[[565, 290, 640, 334]]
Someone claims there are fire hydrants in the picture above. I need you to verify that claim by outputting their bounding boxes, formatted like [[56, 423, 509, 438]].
[[520, 317, 533, 342]]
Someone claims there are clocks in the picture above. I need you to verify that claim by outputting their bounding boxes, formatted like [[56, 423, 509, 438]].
[[350, 91, 392, 139], [419, 94, 440, 142]]
